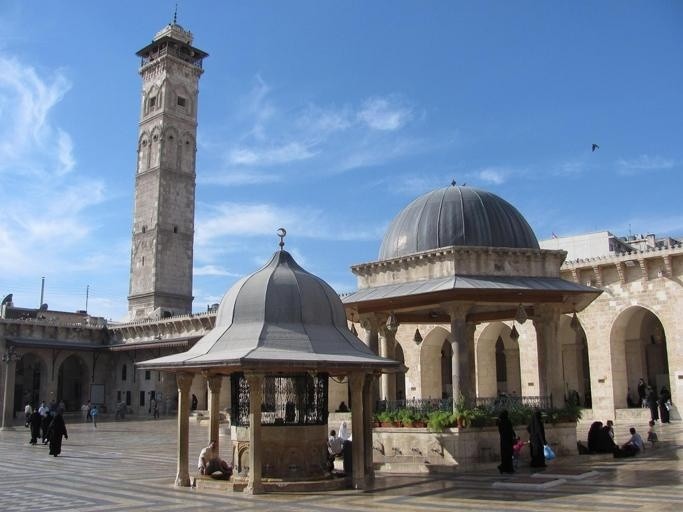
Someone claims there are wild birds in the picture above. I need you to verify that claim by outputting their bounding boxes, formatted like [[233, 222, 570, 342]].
[[552, 232, 559, 239], [450, 179, 456, 186], [591, 144, 599, 153], [461, 182, 466, 186]]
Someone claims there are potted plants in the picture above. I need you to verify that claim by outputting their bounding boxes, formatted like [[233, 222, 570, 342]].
[[370, 393, 581, 434]]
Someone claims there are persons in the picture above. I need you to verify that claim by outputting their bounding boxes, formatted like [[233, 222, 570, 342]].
[[329, 430, 344, 457], [198, 439, 232, 477], [338, 421, 348, 441], [119, 401, 127, 420], [23, 390, 99, 457], [512, 436, 531, 469], [153, 399, 160, 419], [338, 400, 348, 412], [586, 376, 672, 458], [191, 394, 198, 410], [526, 411, 548, 467], [497, 409, 517, 474]]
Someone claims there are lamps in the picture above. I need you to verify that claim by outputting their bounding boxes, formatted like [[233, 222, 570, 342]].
[[385, 300, 399, 337], [509, 320, 519, 343], [513, 292, 528, 324], [411, 318, 423, 346], [569, 302, 580, 333], [348, 312, 357, 338]]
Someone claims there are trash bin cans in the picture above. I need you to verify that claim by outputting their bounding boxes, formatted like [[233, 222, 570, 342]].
[[343, 440, 352, 474]]
